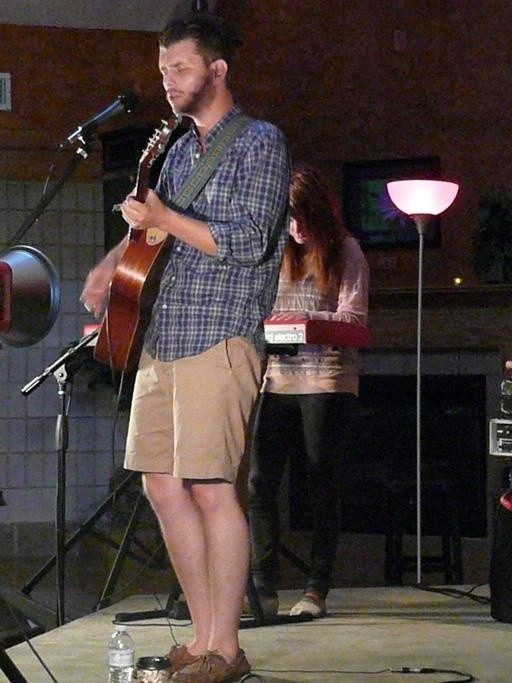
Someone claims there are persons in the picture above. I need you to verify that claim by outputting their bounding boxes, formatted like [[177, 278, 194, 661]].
[[238, 157, 376, 613], [73, 11, 292, 682]]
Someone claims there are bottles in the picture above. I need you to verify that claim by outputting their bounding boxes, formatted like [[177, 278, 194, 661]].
[[106, 625, 135, 683]]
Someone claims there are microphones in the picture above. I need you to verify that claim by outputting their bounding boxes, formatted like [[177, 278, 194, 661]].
[[61, 91, 139, 148]]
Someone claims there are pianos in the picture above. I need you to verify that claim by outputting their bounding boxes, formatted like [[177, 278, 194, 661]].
[[83, 320, 373, 351]]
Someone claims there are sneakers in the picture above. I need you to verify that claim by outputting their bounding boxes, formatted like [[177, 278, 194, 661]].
[[288, 593, 328, 620], [132, 643, 203, 681], [170, 646, 252, 683], [241, 592, 280, 618]]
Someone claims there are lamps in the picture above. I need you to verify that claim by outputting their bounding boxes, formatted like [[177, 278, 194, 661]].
[[388, 180, 460, 586]]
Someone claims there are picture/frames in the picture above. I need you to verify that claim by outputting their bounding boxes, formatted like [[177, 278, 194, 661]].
[[341, 155, 441, 250]]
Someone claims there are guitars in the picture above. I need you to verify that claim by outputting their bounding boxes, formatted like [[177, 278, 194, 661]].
[[93, 114, 179, 372]]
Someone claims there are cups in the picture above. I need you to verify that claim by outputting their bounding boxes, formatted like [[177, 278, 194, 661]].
[[136, 656, 171, 683]]
